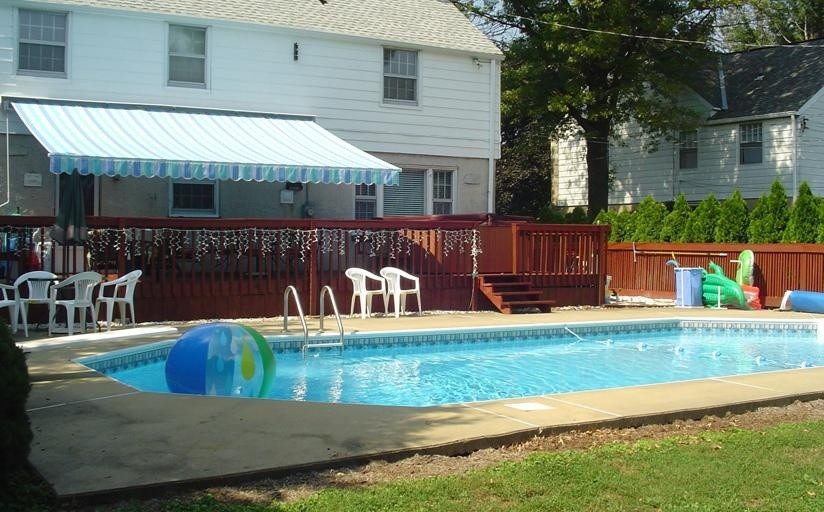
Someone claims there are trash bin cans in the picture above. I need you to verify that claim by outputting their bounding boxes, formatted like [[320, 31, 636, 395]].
[[674, 268, 704, 308]]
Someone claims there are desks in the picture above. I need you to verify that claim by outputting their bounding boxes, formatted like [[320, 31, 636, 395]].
[[28, 278, 101, 334]]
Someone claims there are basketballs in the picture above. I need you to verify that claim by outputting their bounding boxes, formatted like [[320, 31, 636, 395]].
[[166, 324, 275, 399]]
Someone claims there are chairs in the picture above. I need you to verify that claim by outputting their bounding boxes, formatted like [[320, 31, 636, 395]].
[[379, 267, 423, 318], [14, 271, 59, 335], [345, 267, 388, 320], [48, 271, 102, 337], [95, 269, 142, 331], [0, 282, 28, 337]]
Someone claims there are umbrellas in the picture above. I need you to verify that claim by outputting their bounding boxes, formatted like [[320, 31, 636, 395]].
[[49, 169, 89, 286]]
